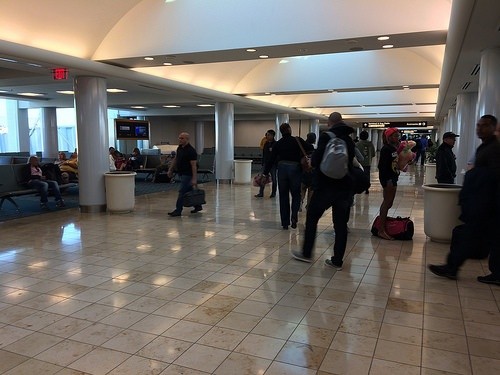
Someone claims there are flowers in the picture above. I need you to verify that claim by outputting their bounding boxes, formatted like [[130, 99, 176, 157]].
[[392, 139, 418, 174]]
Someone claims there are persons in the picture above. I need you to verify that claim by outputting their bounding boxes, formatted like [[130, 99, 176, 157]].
[[375, 128, 400, 216], [435, 132, 456, 184], [289, 112, 355, 271], [155, 150, 175, 183], [167, 132, 203, 216], [415, 135, 430, 166], [23, 156, 65, 210], [427, 115, 500, 284], [109, 147, 142, 171], [253, 123, 376, 233], [55, 151, 69, 174]]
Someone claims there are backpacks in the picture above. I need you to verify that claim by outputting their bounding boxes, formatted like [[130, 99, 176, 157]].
[[43, 163, 61, 185], [319, 131, 350, 181]]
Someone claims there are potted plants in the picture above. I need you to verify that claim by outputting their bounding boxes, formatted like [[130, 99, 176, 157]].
[[423, 139, 438, 185]]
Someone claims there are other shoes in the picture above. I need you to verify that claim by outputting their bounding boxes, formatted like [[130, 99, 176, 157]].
[[191, 207, 202, 213], [291, 250, 313, 263], [291, 223, 297, 228], [40, 203, 49, 211], [56, 200, 66, 209], [477, 271, 500, 286], [427, 262, 459, 280], [283, 225, 288, 229], [270, 194, 275, 198], [324, 257, 342, 271], [168, 209, 181, 216], [254, 193, 263, 197]]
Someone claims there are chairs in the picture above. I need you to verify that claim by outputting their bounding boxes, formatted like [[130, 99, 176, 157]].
[[0, 146, 263, 214]]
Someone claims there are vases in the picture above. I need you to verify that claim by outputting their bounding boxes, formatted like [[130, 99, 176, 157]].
[[103, 170, 138, 216], [234, 159, 253, 185], [420, 183, 468, 243]]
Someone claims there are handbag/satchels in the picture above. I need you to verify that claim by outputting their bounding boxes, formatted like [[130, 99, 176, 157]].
[[301, 155, 312, 171], [183, 186, 206, 207], [370, 215, 414, 240], [253, 174, 272, 187], [352, 165, 372, 195]]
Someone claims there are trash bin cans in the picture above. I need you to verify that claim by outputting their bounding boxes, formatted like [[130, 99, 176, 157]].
[[421, 183, 463, 244], [424, 163, 437, 183], [234, 160, 253, 184], [103, 170, 137, 214]]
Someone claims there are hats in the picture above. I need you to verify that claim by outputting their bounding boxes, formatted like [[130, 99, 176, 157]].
[[384, 128, 397, 137], [443, 132, 459, 139], [359, 131, 368, 141]]
